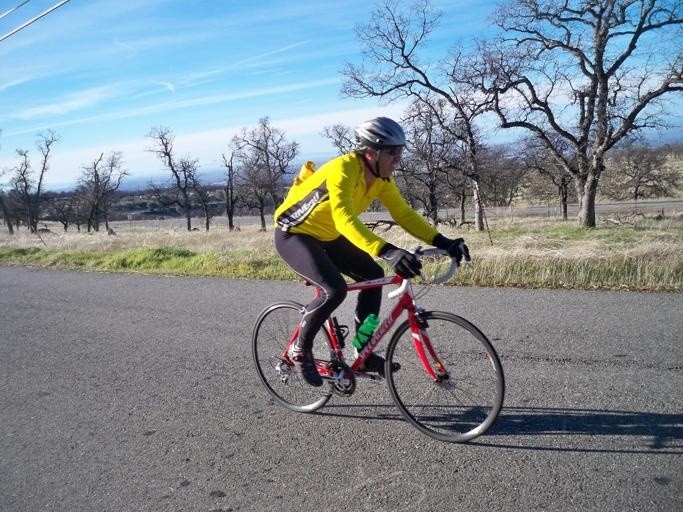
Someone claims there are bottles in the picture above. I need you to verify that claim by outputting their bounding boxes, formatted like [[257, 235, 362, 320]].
[[352, 314, 380, 350]]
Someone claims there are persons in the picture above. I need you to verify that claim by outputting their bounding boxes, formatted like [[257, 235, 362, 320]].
[[271, 116, 470, 386]]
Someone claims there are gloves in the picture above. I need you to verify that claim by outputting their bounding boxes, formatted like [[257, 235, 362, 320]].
[[377, 242, 422, 280], [431, 233, 471, 267]]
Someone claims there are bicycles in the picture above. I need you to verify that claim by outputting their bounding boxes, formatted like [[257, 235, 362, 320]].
[[252, 243, 505, 443]]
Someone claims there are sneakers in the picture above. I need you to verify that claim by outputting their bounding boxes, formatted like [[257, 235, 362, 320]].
[[357, 349, 399, 376], [287, 340, 323, 388]]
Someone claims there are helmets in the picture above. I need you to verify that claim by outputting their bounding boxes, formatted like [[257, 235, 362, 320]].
[[354, 116, 406, 151]]
